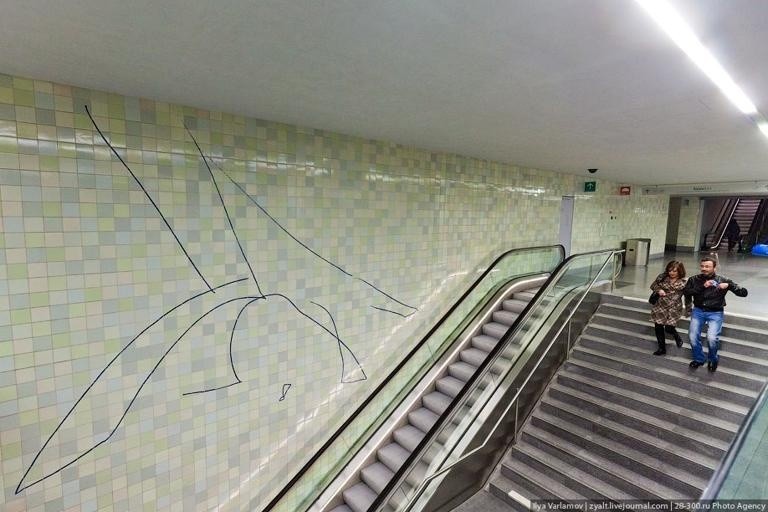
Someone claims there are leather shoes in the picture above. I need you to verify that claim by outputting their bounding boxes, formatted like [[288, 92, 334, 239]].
[[688, 358, 707, 369], [708, 360, 718, 372], [674, 333, 684, 348], [653, 348, 667, 355]]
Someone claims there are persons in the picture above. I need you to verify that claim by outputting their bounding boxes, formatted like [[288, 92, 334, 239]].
[[650, 260, 693, 355], [726, 219, 741, 251], [683, 257, 748, 372]]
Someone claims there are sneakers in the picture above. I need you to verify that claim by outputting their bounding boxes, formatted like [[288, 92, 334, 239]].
[[728, 245, 734, 251]]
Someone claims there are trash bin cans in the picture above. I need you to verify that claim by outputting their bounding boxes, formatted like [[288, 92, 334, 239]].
[[625, 239, 651, 266]]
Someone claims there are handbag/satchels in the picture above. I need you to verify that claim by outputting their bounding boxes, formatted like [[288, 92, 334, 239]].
[[649, 291, 660, 305]]
